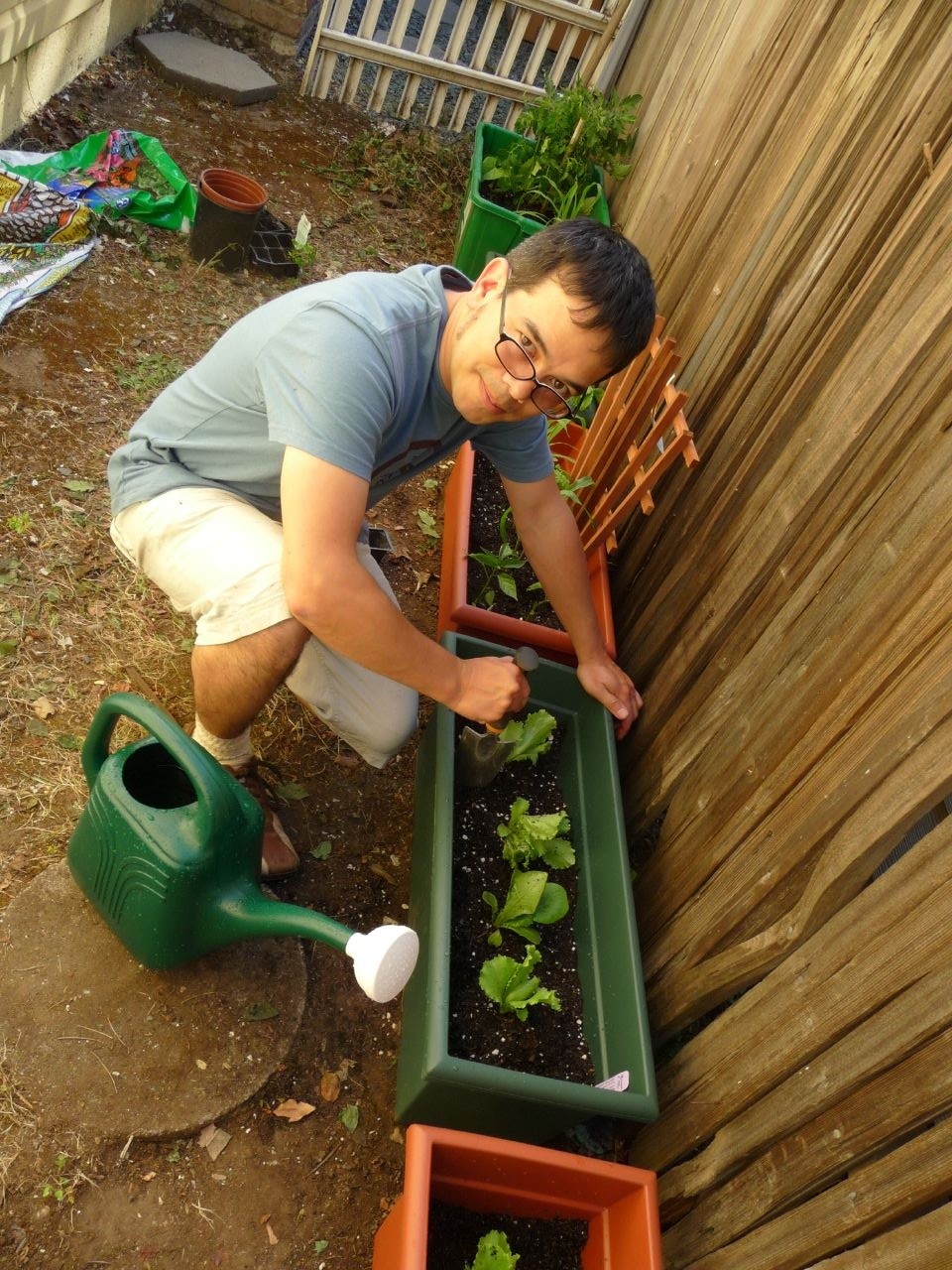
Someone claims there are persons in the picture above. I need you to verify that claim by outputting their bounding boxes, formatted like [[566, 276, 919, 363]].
[[108, 217, 654, 878]]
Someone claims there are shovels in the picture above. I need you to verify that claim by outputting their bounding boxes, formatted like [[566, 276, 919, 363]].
[[458, 645, 541, 794]]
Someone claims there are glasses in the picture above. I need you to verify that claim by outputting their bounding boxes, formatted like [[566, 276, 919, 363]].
[[494, 266, 587, 420]]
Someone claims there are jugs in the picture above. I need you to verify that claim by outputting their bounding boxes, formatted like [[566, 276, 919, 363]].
[[66, 691, 419, 1004]]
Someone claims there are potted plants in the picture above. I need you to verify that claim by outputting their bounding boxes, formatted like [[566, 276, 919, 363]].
[[372, 1124, 664, 1270], [397, 632, 660, 1146], [438, 404, 615, 660], [453, 87, 638, 277]]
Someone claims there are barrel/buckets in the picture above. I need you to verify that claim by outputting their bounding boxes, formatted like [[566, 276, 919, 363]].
[[194, 168, 267, 273]]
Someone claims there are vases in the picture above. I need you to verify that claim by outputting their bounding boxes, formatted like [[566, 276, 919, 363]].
[[192, 168, 268, 274]]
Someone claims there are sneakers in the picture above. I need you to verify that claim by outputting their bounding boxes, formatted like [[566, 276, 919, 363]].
[[182, 721, 300, 878]]
